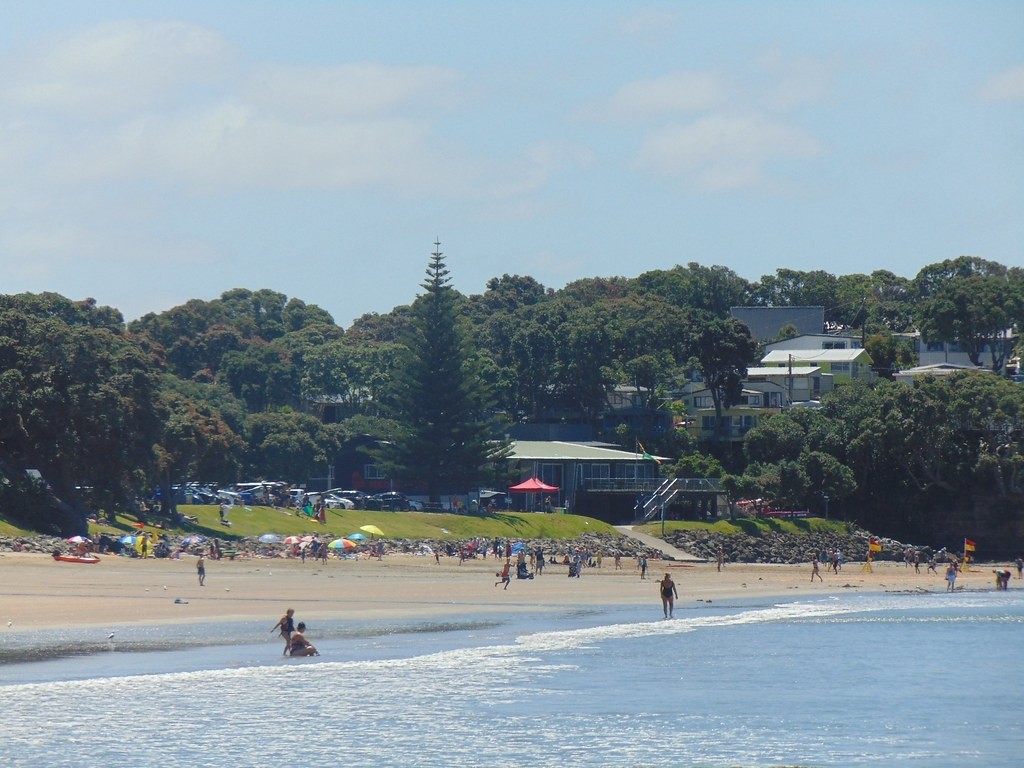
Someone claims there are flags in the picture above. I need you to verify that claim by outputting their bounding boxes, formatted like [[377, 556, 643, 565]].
[[869, 539, 881, 551], [966, 540, 976, 551]]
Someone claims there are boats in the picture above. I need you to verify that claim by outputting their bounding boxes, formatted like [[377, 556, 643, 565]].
[[52, 549, 101, 564]]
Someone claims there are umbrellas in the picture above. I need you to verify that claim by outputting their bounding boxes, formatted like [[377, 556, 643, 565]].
[[118, 536, 152, 554], [259, 534, 323, 544], [182, 535, 206, 544], [68, 536, 92, 543], [328, 524, 384, 550]]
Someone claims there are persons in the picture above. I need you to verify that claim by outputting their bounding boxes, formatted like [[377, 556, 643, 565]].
[[139, 497, 146, 512], [435, 536, 648, 590], [272, 609, 317, 656], [77, 531, 101, 556], [368, 539, 383, 561], [139, 536, 180, 559], [717, 547, 723, 571], [219, 503, 225, 520], [992, 557, 1023, 590], [810, 547, 842, 583], [209, 538, 219, 557], [299, 536, 327, 565], [287, 493, 326, 523], [196, 554, 206, 586], [904, 550, 958, 592], [660, 573, 678, 618]]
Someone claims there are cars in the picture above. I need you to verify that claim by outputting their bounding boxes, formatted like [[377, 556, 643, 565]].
[[336, 491, 382, 511], [305, 492, 340, 508], [151, 483, 305, 505], [373, 492, 410, 513], [326, 494, 355, 510], [409, 500, 425, 512]]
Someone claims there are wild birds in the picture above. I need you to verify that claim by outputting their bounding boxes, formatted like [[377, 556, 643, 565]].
[[7, 619, 12, 627], [108, 631, 114, 639]]
